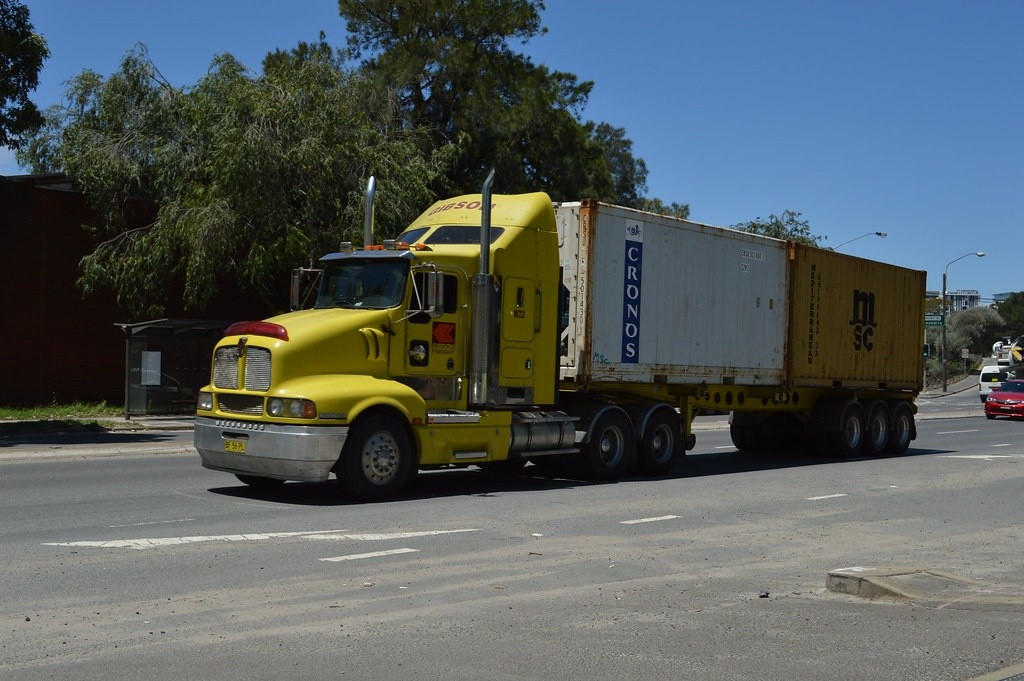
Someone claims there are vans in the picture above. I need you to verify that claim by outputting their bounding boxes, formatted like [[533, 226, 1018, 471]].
[[978, 365, 1015, 403]]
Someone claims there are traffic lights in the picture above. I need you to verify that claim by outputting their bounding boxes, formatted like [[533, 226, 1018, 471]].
[[923, 343, 929, 358]]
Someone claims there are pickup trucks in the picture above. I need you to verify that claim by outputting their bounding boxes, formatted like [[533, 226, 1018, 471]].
[[997, 345, 1012, 365]]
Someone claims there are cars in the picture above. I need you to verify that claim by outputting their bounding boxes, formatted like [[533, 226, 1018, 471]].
[[984, 379, 1024, 421]]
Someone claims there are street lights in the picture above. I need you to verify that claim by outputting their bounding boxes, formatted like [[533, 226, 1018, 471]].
[[829, 232, 889, 253], [942, 250, 986, 390]]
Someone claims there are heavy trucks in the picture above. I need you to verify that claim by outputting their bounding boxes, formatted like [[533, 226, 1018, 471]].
[[191, 167, 928, 499]]
